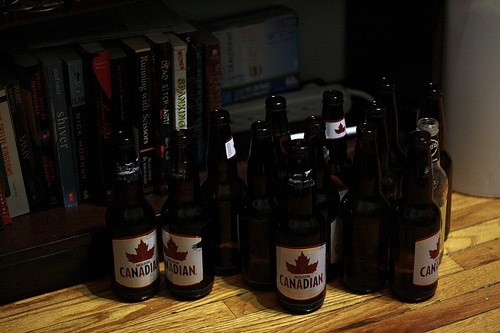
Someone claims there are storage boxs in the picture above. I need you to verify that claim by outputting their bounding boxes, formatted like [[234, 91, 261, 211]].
[[207, 5, 302, 107]]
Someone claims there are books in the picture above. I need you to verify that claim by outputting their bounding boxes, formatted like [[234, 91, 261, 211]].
[[0, 0, 300, 225]]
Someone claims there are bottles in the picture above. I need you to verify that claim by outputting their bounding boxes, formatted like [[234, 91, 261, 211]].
[[159, 128, 214, 301], [199, 108, 246, 278], [103, 129, 159, 302], [242, 77, 453, 315]]
